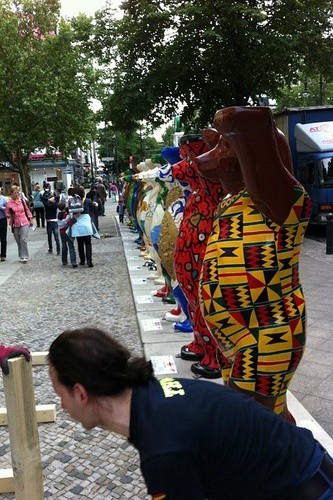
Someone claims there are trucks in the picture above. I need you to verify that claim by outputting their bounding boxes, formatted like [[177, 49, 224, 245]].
[[273, 104, 332, 228]]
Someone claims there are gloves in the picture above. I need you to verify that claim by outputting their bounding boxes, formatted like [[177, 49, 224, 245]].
[[0, 345, 30, 375]]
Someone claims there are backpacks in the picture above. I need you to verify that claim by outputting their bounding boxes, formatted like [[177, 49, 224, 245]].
[[68, 197, 84, 212], [43, 189, 51, 198]]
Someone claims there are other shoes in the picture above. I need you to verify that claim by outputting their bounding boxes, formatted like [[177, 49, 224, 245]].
[[88, 263, 94, 267], [79, 262, 85, 265], [19, 258, 27, 263], [1, 257, 5, 261], [72, 264, 78, 267]]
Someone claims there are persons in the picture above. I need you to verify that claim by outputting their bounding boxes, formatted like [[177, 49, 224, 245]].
[[31, 173, 129, 268], [12, 181, 29, 204], [47, 327, 333, 500], [5, 185, 34, 263], [0, 186, 8, 261]]
